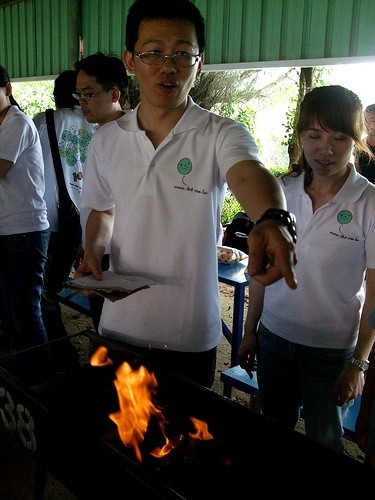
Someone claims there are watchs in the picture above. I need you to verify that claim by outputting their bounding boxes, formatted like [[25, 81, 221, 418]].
[[349, 358, 369, 371]]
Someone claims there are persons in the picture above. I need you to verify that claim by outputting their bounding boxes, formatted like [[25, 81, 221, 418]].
[[0, 64, 53, 389], [32, 71, 99, 375], [74, 0, 297, 389], [72, 51, 134, 130], [237, 85, 375, 456], [355, 104, 375, 186]]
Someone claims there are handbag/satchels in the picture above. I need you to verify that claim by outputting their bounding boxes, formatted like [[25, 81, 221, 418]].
[[58, 191, 82, 260]]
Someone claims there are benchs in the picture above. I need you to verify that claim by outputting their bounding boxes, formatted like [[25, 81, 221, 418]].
[[57, 276, 90, 318], [220, 356, 363, 443]]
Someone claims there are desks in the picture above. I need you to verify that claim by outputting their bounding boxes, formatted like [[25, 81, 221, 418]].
[[218, 256, 249, 369]]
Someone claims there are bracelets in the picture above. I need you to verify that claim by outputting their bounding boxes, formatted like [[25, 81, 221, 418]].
[[255, 208, 296, 227], [255, 212, 297, 245]]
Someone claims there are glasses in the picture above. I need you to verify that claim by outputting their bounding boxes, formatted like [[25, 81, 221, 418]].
[[71, 88, 117, 101], [131, 52, 200, 67]]
